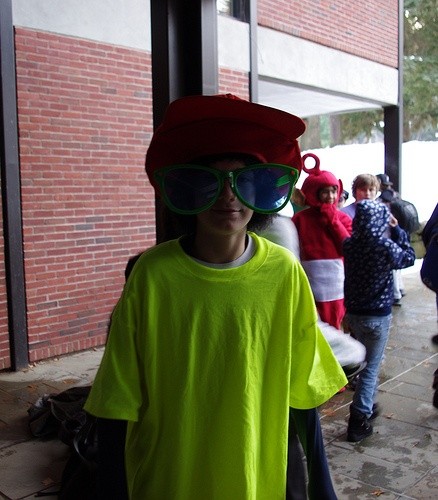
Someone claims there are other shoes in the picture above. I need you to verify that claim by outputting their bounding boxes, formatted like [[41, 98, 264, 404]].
[[348, 403, 382, 421], [347, 424, 374, 442]]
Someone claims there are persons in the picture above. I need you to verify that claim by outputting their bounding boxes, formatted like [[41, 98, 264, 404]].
[[335, 173, 438, 443], [84, 94, 350, 500]]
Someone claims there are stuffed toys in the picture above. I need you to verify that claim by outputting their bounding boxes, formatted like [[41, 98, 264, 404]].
[[291, 154, 353, 391]]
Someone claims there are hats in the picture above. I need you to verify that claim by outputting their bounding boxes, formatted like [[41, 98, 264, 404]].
[[378, 189, 398, 200], [344, 189, 349, 200], [144, 94, 305, 196], [377, 174, 393, 186]]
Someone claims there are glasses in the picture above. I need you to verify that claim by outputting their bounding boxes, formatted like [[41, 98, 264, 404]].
[[339, 197, 344, 202], [155, 163, 299, 215]]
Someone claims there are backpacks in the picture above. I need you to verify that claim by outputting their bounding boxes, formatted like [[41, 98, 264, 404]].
[[389, 199, 421, 233]]
